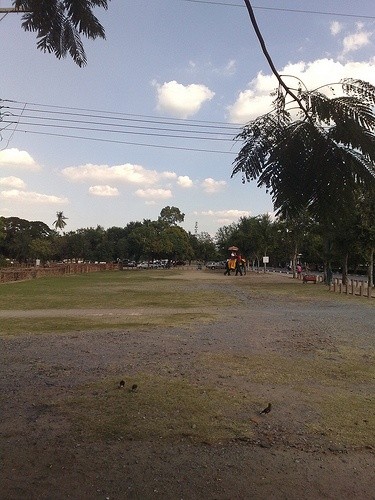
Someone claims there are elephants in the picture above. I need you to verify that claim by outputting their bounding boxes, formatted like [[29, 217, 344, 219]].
[[223, 258, 247, 277]]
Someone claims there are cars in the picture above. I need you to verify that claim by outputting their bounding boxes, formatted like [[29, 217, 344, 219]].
[[122, 259, 174, 270], [303, 273, 317, 284], [206, 260, 227, 270], [56, 258, 88, 264]]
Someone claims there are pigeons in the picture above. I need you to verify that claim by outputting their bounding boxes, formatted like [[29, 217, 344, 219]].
[[131, 383, 138, 391], [258, 402, 272, 416], [119, 379, 126, 389]]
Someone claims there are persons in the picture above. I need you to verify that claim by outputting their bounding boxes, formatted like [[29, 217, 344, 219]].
[[223, 259, 247, 276], [296, 264, 302, 279]]
